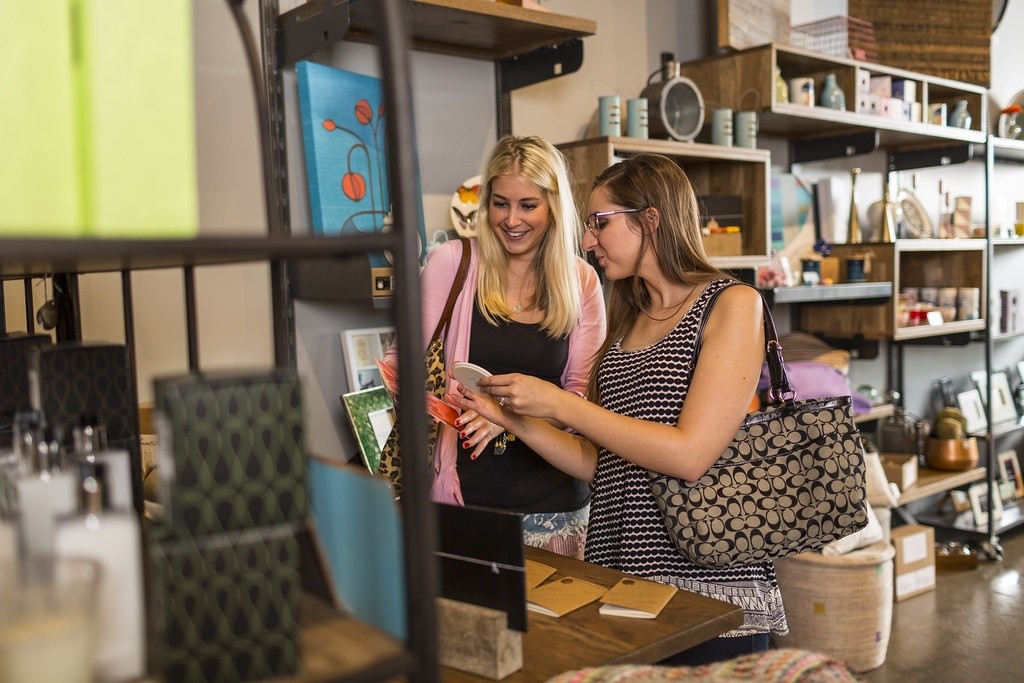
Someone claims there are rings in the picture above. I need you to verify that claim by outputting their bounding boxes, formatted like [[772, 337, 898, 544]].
[[498, 397, 504, 408]]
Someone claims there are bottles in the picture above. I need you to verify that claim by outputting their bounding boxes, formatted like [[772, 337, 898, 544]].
[[949, 100, 972, 130], [0, 410, 145, 683], [801, 258, 821, 286], [847, 255, 867, 283], [821, 75, 846, 111]]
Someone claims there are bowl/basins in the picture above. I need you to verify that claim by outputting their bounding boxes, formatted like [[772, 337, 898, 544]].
[[924, 435, 979, 472]]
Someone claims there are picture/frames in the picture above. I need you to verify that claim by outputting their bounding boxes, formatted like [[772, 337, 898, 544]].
[[997, 449, 1024, 500], [977, 372, 1018, 424], [340, 386, 406, 477], [970, 368, 987, 405], [938, 376, 959, 415], [969, 483, 1003, 525], [957, 388, 986, 432]]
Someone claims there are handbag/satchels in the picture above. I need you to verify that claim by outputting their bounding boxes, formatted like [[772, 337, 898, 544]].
[[380, 239, 472, 492], [649, 283, 869, 569]]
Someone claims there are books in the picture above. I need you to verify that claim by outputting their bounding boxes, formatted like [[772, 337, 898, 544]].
[[599, 577, 679, 619], [523, 559, 557, 589], [525, 576, 609, 619]]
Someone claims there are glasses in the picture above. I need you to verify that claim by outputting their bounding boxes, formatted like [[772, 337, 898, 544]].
[[584, 208, 644, 239]]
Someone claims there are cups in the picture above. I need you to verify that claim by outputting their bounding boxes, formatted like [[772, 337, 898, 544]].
[[898, 287, 980, 328], [791, 78, 815, 107]]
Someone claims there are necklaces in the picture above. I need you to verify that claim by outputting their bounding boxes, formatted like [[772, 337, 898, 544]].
[[513, 305, 522, 315]]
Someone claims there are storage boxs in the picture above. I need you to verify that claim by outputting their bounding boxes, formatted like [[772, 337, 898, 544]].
[[873, 451, 919, 491], [887, 525, 937, 603]]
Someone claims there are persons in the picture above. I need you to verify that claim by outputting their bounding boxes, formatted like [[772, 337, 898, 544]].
[[448, 153, 788, 661], [381, 134, 608, 566]]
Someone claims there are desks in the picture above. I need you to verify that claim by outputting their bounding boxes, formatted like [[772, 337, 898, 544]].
[[382, 545, 741, 683]]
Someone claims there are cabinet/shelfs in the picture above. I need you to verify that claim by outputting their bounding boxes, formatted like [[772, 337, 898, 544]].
[[277, 1, 1024, 588]]
